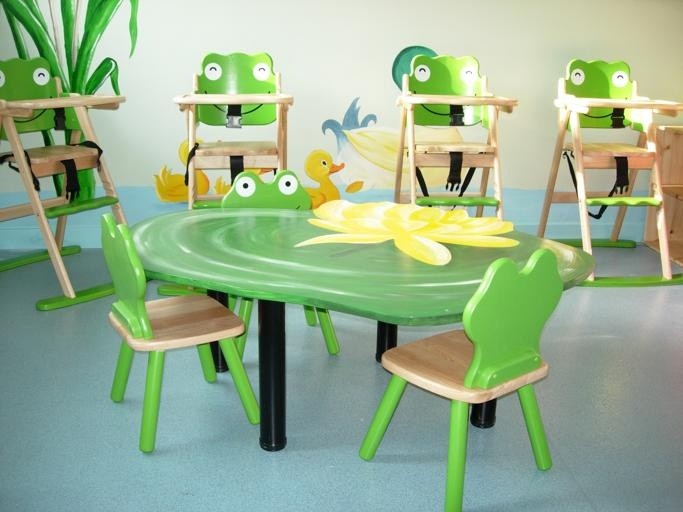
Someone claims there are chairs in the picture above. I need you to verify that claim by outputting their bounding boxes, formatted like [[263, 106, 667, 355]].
[[395, 54, 518, 221], [0, 56, 151, 309], [536, 59, 676, 280], [179, 52, 292, 210]]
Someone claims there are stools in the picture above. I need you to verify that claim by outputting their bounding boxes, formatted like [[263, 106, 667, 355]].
[[361, 249, 565, 510], [99, 214, 262, 451], [222, 171, 338, 364]]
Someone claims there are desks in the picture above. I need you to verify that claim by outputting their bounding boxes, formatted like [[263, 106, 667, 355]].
[[127, 204, 595, 451]]
